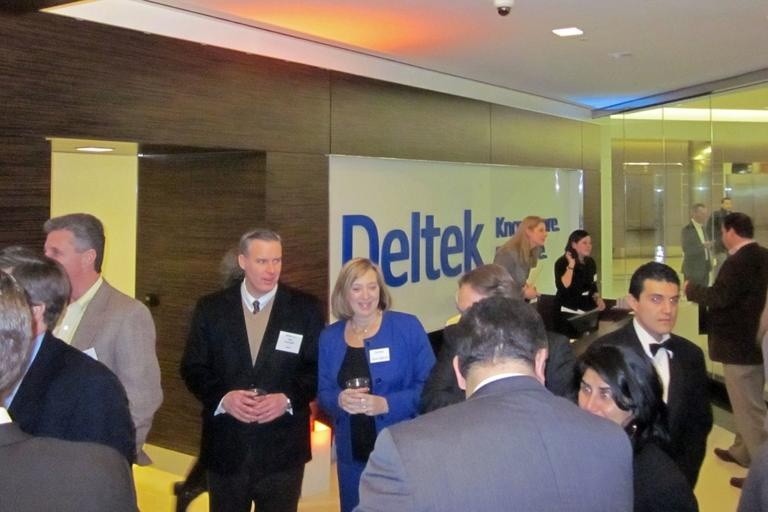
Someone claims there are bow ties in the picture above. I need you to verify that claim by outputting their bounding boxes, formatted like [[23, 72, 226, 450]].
[[650, 338, 675, 357]]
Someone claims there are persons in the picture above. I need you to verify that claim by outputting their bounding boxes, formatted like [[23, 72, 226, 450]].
[[0, 262, 139, 511], [45, 214, 164, 466], [0, 242, 137, 467]]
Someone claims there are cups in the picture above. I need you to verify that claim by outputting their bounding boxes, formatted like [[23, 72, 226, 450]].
[[246, 388, 268, 401], [345, 376, 371, 392]]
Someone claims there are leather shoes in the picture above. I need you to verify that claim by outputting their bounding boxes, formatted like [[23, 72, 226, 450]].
[[731, 477, 747, 488], [174, 481, 193, 512], [715, 448, 746, 468]]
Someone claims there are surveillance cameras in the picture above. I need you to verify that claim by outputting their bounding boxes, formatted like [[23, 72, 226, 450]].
[[495, 0, 513, 16]]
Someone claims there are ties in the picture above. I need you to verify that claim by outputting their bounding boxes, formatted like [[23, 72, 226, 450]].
[[701, 226, 710, 261], [252, 301, 260, 315]]
